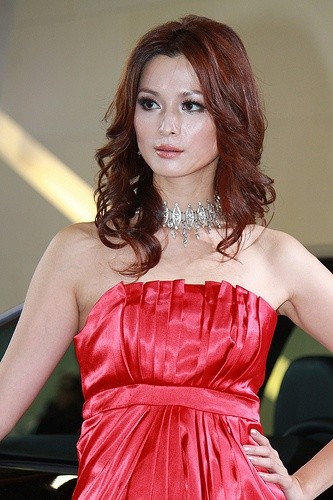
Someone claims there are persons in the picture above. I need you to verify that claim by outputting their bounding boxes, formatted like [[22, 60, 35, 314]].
[[0, 14, 333, 500]]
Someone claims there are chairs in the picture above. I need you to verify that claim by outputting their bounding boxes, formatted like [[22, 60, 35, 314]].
[[273, 354, 333, 476]]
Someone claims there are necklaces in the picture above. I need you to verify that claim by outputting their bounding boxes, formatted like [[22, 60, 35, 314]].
[[158, 196, 234, 248]]
